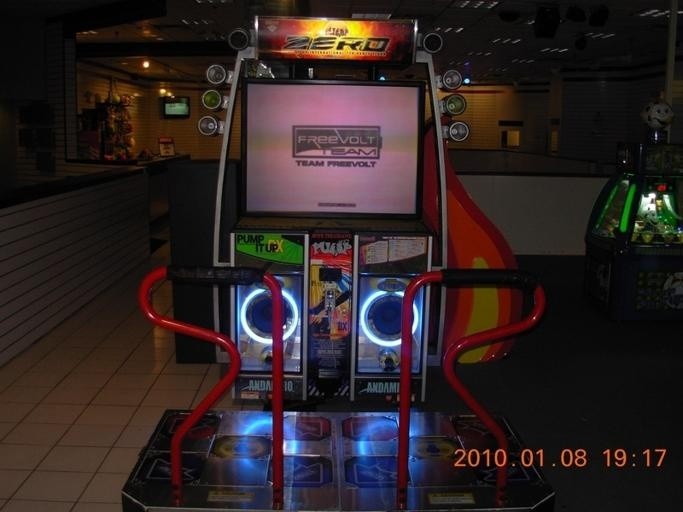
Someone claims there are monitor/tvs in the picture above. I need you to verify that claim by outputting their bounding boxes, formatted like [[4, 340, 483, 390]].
[[241, 77, 426, 221], [164, 97, 190, 116]]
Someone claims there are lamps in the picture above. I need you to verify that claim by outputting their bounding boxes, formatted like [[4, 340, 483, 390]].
[[421, 29, 469, 144], [196, 28, 249, 138]]
